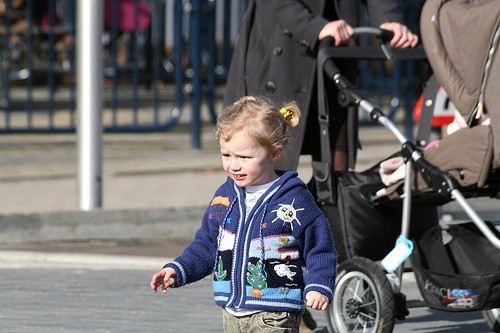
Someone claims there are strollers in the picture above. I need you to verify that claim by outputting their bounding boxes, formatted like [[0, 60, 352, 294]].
[[309, 27, 500, 332]]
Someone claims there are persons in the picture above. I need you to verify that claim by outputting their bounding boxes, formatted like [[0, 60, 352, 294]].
[[221, 1, 419, 333], [151, 96, 337, 333]]
